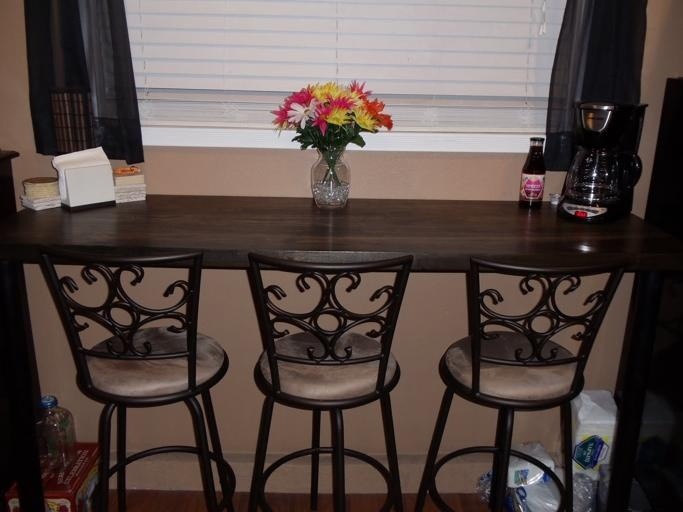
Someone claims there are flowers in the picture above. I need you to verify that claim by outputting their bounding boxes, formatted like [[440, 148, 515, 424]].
[[271, 80, 394, 148]]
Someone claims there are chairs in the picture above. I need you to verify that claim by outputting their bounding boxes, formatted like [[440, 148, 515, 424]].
[[38, 244, 237, 512], [415, 256, 624, 511], [242, 251, 413, 512]]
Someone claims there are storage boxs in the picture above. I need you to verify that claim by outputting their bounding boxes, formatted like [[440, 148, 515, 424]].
[[5, 442, 102, 512]]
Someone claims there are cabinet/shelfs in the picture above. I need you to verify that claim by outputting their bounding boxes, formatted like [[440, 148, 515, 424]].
[[0, 150, 47, 511], [611, 75, 683, 512]]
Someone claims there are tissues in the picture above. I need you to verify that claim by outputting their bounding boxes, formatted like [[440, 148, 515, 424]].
[[53, 147, 117, 212]]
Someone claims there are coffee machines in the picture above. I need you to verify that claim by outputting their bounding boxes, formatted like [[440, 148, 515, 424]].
[[556, 102, 649, 225]]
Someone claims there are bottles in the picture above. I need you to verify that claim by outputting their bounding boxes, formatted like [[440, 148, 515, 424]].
[[38, 395, 78, 473], [519, 137, 546, 209]]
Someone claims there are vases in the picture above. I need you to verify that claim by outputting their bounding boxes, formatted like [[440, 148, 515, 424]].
[[309, 147, 353, 209]]
